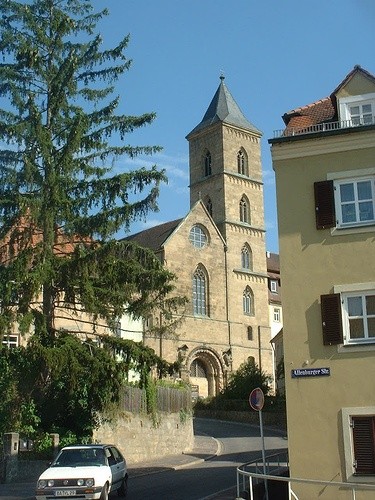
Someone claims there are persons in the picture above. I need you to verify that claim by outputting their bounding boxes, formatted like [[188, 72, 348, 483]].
[[89, 450, 105, 465]]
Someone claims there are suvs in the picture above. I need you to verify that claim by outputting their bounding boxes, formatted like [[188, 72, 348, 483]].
[[34, 443, 129, 500]]
